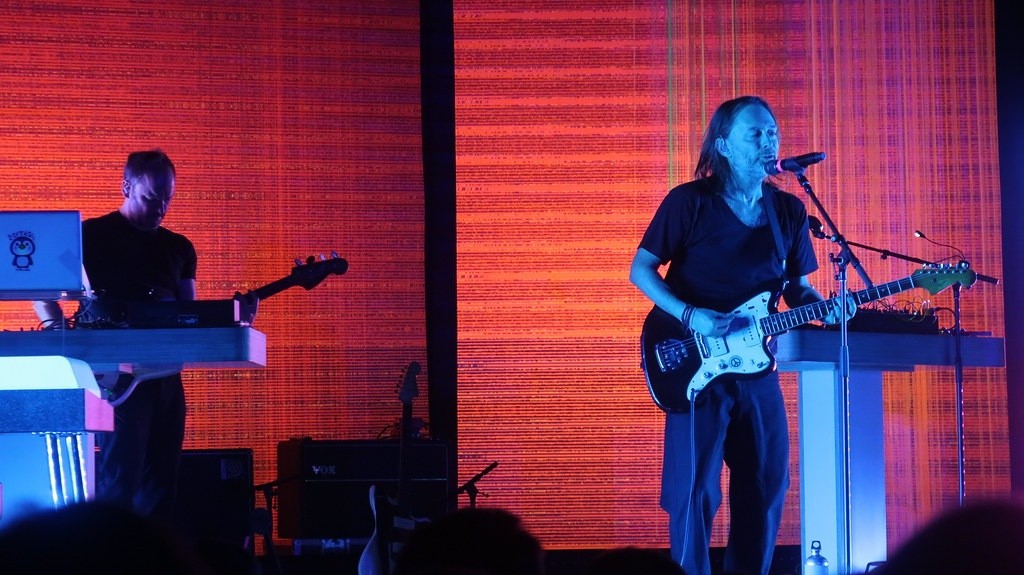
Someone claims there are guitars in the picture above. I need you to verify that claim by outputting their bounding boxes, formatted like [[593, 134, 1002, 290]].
[[357, 359, 448, 575], [70, 250, 349, 405], [639, 258, 977, 414]]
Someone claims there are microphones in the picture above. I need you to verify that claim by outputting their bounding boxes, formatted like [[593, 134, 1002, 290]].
[[765, 151, 826, 175]]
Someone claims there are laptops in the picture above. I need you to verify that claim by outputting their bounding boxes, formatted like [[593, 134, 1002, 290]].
[[0, 210, 107, 304]]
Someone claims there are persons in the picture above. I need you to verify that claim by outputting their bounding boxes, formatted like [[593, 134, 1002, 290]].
[[35, 152, 196, 503], [0, 502, 1024, 575], [629, 95, 856, 575]]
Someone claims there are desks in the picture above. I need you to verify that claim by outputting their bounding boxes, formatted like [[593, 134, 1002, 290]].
[[0, 328, 266, 501], [770, 329, 1006, 575]]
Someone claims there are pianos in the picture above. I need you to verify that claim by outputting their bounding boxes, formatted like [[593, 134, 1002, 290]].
[[772, 330, 1006, 372], [0, 326, 269, 370]]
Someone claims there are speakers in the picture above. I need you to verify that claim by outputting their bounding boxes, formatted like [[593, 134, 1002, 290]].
[[93, 450, 252, 558], [276, 441, 450, 543]]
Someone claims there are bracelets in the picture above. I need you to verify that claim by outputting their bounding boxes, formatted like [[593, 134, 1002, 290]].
[[681, 304, 696, 331]]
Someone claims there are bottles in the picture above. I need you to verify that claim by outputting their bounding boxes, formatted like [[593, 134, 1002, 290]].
[[805, 540, 829, 575]]
